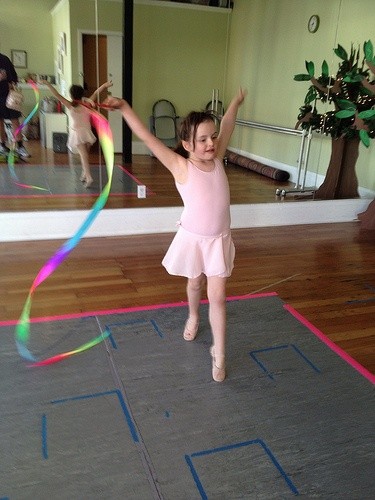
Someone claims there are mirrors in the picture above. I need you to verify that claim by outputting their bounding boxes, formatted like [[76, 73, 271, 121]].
[[0, 0, 375, 212]]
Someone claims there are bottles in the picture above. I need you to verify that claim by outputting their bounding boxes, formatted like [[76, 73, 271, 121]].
[[58, 100, 61, 113]]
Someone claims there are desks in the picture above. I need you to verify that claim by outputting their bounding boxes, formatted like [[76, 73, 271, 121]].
[[16, 81, 57, 91]]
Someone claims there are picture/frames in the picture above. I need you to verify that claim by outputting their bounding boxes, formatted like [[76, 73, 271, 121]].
[[56, 52, 63, 75], [60, 32, 65, 57], [10, 48, 28, 69]]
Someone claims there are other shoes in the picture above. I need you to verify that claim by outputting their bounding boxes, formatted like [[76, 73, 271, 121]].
[[84, 178, 94, 188], [209, 345, 225, 382], [184, 315, 200, 340], [16, 147, 31, 158], [0, 144, 5, 152], [80, 171, 85, 181]]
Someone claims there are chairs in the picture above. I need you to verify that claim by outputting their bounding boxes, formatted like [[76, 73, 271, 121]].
[[147, 96, 178, 159], [204, 96, 226, 122]]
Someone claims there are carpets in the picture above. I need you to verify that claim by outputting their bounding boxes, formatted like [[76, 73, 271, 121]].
[[0, 158, 158, 199], [0, 293, 375, 500]]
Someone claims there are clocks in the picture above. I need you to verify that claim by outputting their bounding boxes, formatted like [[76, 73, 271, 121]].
[[307, 14, 319, 32]]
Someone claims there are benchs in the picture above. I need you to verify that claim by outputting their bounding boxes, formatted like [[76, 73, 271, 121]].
[[38, 108, 69, 150]]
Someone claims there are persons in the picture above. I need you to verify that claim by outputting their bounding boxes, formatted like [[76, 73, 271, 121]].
[[0, 52, 31, 158], [37, 79, 113, 188], [99, 86, 248, 383]]
